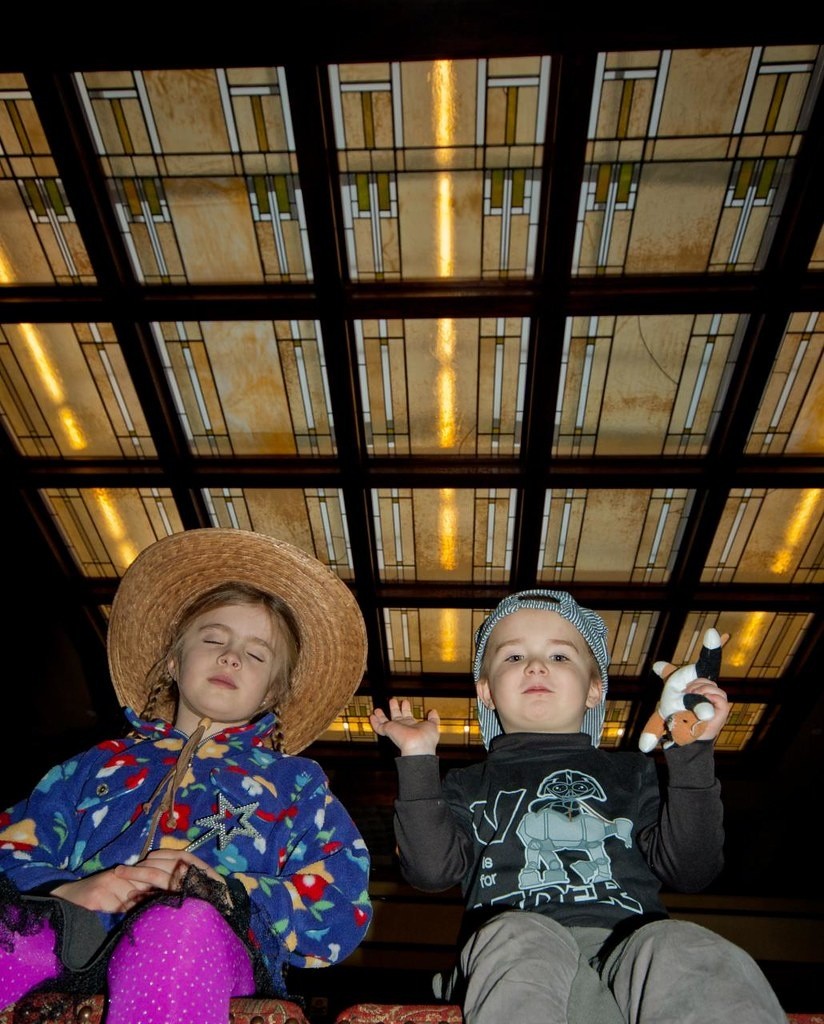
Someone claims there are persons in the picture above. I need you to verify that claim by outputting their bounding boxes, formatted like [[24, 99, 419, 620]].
[[368, 589, 792, 1024], [0, 528, 374, 1024]]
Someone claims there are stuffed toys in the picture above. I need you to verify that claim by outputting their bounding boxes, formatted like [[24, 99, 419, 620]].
[[638, 628, 723, 755]]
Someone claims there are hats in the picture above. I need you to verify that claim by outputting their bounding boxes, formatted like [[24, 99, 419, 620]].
[[107, 527, 370, 753], [473, 589, 609, 751]]
